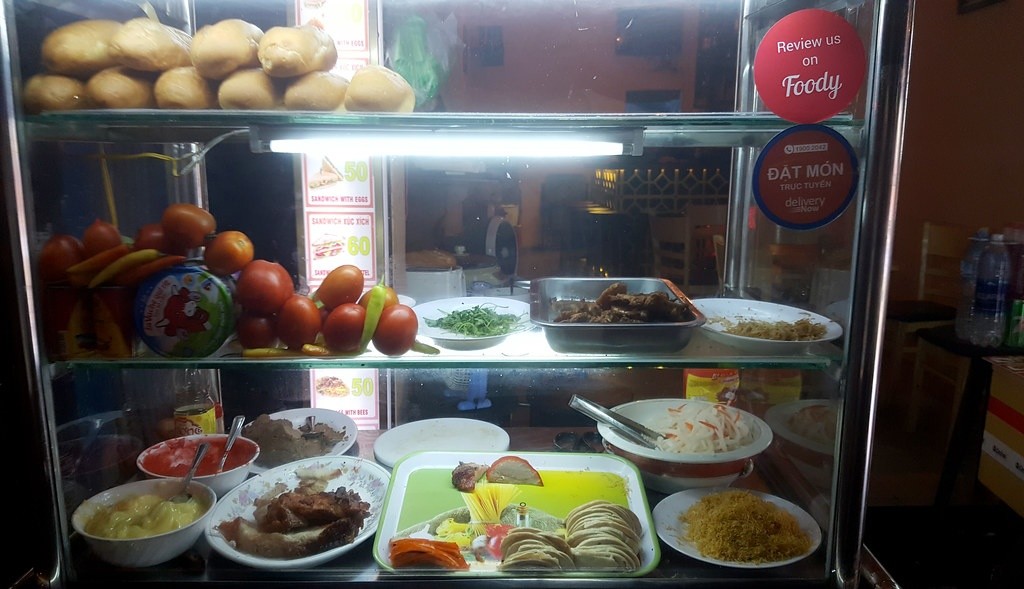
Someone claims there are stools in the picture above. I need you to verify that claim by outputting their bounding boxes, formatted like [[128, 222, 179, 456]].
[[567, 199, 618, 274]]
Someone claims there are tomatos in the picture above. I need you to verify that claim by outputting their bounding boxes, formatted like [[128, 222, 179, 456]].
[[42, 202, 419, 358]]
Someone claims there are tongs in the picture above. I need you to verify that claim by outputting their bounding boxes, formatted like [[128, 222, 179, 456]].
[[566, 393, 668, 450]]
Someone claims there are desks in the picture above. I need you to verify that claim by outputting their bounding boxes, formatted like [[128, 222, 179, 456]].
[[402, 249, 498, 271]]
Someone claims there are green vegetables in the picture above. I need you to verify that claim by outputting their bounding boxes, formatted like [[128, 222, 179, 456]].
[[420, 302, 532, 338]]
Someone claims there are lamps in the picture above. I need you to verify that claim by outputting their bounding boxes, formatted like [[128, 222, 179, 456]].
[[252, 127, 649, 167]]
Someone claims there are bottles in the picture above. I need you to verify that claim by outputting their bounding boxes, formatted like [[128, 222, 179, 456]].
[[970, 234, 1010, 348], [954, 226, 990, 341], [134, 246, 241, 360], [173, 368, 217, 436]]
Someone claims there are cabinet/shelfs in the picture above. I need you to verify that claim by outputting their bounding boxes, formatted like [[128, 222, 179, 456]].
[[0, 0, 919, 589]]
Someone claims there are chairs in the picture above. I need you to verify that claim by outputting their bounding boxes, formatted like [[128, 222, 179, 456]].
[[903, 320, 1009, 466], [707, 234, 774, 303], [766, 242, 825, 308], [461, 215, 518, 283], [878, 220, 982, 414], [653, 215, 715, 296]]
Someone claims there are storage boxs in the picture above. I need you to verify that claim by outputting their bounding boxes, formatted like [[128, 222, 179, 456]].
[[530, 276, 706, 352], [978, 360, 1024, 517]]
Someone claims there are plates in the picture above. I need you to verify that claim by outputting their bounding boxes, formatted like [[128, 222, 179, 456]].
[[689, 298, 843, 352], [374, 418, 510, 470], [241, 408, 358, 474], [415, 320, 501, 339], [652, 487, 822, 569], [205, 455, 391, 571]]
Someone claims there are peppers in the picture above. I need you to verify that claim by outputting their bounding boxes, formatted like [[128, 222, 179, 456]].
[[222, 337, 440, 356], [65, 243, 130, 273], [87, 249, 168, 288], [113, 255, 187, 283]]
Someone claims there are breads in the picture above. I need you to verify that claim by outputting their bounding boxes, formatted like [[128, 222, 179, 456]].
[[21, 16, 416, 116]]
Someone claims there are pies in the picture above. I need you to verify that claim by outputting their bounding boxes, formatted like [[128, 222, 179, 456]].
[[498, 499, 643, 571]]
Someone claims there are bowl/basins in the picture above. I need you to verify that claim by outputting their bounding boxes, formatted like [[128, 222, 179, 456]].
[[137, 433, 261, 501], [39, 434, 143, 522], [596, 398, 774, 494], [71, 478, 217, 568], [764, 399, 838, 489]]
